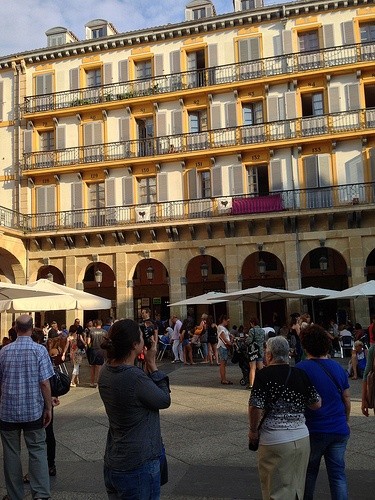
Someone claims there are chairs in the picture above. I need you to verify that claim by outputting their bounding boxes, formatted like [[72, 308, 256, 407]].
[[156, 340, 172, 361], [341, 336, 353, 356]]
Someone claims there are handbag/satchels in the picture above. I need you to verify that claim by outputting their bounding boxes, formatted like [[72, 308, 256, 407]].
[[199, 324, 208, 343], [49, 370, 71, 396], [366, 345, 375, 409], [195, 322, 203, 335], [225, 343, 234, 357], [247, 327, 262, 362], [286, 325, 297, 348], [248, 430, 260, 451], [76, 334, 85, 350]]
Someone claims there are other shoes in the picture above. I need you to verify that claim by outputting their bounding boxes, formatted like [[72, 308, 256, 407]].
[[221, 380, 233, 385], [76, 381, 80, 385], [246, 386, 253, 390], [183, 359, 222, 367], [170, 359, 180, 363], [70, 383, 77, 387]]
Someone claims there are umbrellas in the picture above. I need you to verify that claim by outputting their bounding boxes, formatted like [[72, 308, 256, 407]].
[[166, 279, 375, 327], [1, 279, 110, 321]]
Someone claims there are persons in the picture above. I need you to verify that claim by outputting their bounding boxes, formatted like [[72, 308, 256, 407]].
[[293, 339, 351, 500], [247, 336, 321, 500], [361, 323, 375, 418], [97, 319, 172, 500], [0, 315, 55, 500], [0, 319, 375, 387]]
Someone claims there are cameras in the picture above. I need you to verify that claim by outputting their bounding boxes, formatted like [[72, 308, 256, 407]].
[[139, 323, 155, 349]]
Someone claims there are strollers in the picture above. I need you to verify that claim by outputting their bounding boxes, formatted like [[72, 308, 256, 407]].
[[231, 338, 250, 386]]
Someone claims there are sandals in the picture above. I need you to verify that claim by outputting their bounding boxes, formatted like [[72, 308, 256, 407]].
[[49, 466, 57, 477], [23, 473, 30, 483]]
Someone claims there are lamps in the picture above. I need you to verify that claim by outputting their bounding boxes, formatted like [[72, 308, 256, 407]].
[[46, 271, 54, 282], [257, 259, 266, 280], [146, 266, 154, 286], [199, 262, 208, 282], [95, 268, 103, 287], [319, 256, 328, 277]]
